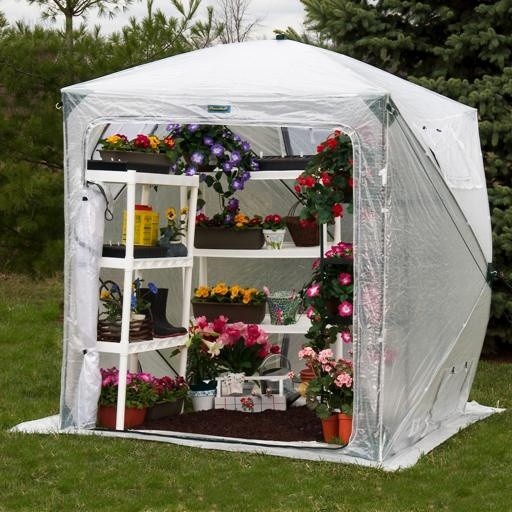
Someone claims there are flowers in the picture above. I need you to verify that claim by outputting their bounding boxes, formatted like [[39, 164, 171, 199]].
[[97, 134, 176, 154], [191, 280, 266, 303], [184, 316, 279, 389], [298, 242, 393, 418], [196, 210, 283, 230], [288, 131, 381, 224], [95, 369, 188, 407], [156, 207, 189, 248], [97, 273, 158, 313], [166, 124, 258, 206]]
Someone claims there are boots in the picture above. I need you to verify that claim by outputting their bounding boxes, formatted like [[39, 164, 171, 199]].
[[137, 287, 188, 337]]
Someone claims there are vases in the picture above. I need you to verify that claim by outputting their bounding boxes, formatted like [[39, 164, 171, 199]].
[[193, 223, 264, 250], [144, 396, 184, 422], [178, 145, 222, 173], [165, 240, 188, 257], [317, 409, 354, 445], [285, 214, 320, 248], [190, 298, 266, 325], [261, 229, 286, 250], [96, 148, 171, 168], [187, 389, 215, 413], [266, 288, 301, 324], [98, 401, 147, 429]]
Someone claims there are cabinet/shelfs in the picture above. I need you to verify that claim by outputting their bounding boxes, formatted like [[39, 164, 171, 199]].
[[181, 168, 354, 400], [84, 168, 200, 432]]
